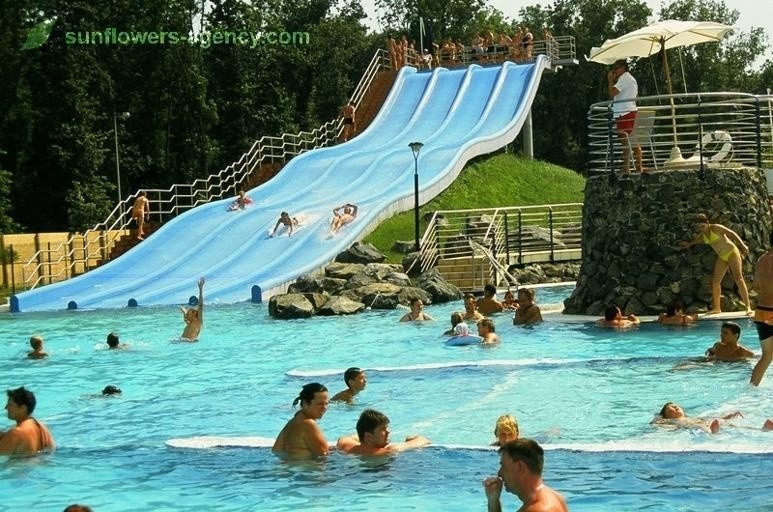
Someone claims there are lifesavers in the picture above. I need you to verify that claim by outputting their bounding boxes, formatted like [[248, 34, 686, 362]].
[[446, 332, 483, 347], [694, 131, 732, 162]]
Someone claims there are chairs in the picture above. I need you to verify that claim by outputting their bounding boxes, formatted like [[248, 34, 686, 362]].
[[605, 111, 658, 172]]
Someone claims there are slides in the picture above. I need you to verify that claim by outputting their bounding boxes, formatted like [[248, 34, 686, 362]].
[[10, 54, 552, 313]]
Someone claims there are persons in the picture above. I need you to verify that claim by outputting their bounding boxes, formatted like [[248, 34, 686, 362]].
[[102, 384, 121, 395], [228, 190, 252, 211], [597, 211, 773, 435], [328, 367, 367, 403], [383, 26, 552, 71], [63, 503, 92, 512], [325, 203, 358, 237], [180, 279, 205, 340], [483, 438, 569, 511], [489, 415, 519, 446], [0, 387, 55, 455], [271, 384, 330, 456], [132, 190, 150, 241], [27, 335, 48, 357], [607, 59, 643, 173], [343, 101, 357, 142], [398, 285, 543, 346], [106, 332, 128, 349], [270, 212, 306, 238], [337, 409, 431, 457]]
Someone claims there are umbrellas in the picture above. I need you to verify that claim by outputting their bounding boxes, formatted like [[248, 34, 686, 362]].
[[586, 19, 734, 144]]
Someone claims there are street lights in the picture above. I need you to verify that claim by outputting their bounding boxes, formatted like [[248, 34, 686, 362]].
[[409, 142, 424, 251], [112, 112, 131, 229]]
